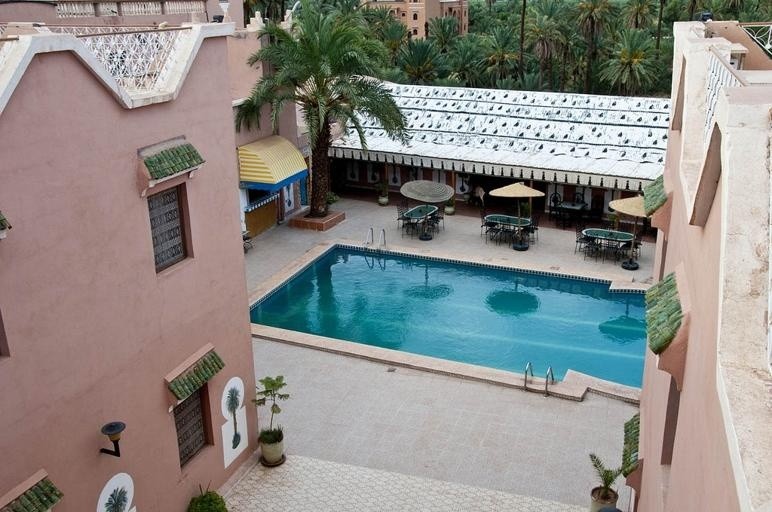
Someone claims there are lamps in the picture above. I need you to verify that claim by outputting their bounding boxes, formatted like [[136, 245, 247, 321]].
[[97, 422, 126, 457]]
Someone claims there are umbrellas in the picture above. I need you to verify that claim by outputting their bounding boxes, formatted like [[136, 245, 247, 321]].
[[488, 181, 545, 241], [608, 193, 652, 259], [398, 180, 455, 232]]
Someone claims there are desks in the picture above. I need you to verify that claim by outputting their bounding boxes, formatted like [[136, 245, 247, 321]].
[[555, 200, 588, 228]]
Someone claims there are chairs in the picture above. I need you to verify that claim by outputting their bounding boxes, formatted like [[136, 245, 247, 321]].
[[548, 192, 584, 230], [587, 195, 604, 224], [573, 220, 643, 266], [396, 197, 445, 240], [479, 204, 541, 248]]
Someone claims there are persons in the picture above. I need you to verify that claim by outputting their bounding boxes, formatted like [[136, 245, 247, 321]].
[[474, 186, 487, 221], [532, 198, 542, 231], [521, 200, 531, 218]]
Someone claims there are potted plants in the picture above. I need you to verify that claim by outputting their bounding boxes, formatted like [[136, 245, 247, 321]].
[[374, 178, 389, 206], [444, 199, 455, 215], [246, 375, 289, 464], [606, 213, 619, 230], [586, 452, 632, 512]]
